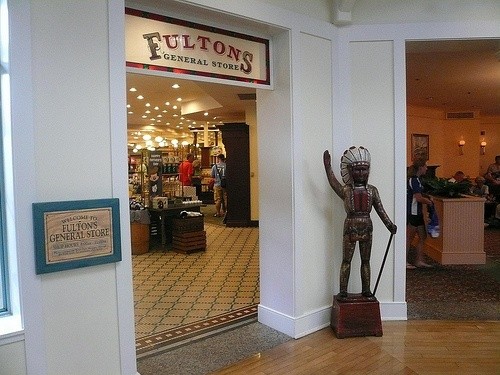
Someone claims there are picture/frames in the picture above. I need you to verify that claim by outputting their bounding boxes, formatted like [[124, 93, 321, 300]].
[[411, 134, 429, 160]]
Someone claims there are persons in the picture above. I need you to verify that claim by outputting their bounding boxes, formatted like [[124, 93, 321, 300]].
[[323, 146, 397, 302], [177, 153, 194, 186], [406, 158, 433, 268], [454, 156, 500, 195], [211, 154, 227, 217]]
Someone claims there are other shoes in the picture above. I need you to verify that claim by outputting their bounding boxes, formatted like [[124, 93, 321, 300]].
[[417, 262, 431, 268], [407, 263, 416, 269], [213, 213, 221, 217]]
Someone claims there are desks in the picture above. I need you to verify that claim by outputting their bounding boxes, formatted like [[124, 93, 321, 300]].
[[485, 200, 498, 228]]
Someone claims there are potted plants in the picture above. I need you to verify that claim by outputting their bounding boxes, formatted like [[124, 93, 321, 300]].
[[423, 177, 473, 198]]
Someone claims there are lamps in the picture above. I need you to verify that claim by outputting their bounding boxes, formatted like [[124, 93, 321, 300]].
[[480, 137, 487, 154], [458, 137, 466, 154]]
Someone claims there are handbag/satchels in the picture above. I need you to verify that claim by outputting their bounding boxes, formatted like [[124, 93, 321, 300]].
[[221, 179, 227, 188]]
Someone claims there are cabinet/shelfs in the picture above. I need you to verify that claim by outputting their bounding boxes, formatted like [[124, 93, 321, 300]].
[[128, 144, 219, 203]]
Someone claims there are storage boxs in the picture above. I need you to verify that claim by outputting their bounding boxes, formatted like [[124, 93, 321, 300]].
[[170, 215, 206, 255]]
